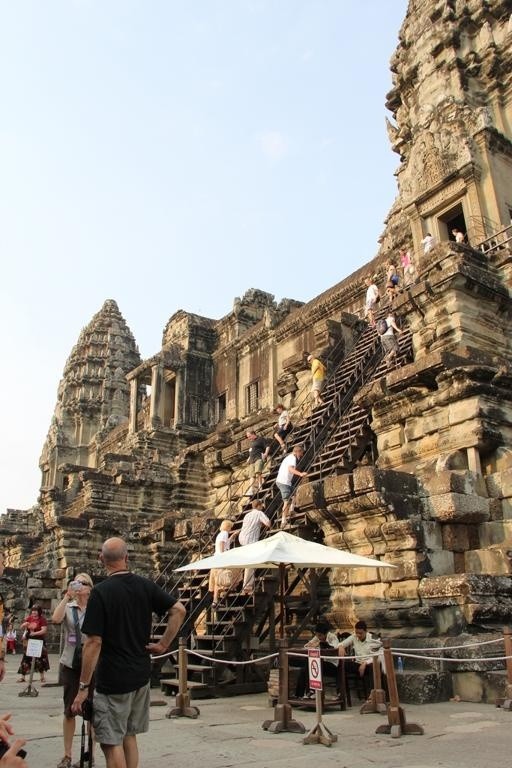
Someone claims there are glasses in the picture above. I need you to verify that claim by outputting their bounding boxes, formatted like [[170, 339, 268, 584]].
[[78, 579, 90, 585]]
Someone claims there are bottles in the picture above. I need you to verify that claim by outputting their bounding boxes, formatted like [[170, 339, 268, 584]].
[[397, 657, 404, 673]]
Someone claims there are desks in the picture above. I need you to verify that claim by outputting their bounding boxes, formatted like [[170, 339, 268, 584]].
[[279, 649, 346, 714]]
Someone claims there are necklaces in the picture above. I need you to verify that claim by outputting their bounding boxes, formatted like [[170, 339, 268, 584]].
[[108, 569, 130, 576]]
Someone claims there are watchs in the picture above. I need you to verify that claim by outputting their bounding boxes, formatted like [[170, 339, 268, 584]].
[[79, 681, 90, 689]]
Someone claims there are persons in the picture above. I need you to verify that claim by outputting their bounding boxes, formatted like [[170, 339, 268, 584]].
[[338, 621, 390, 704], [52, 573, 99, 768], [238, 499, 271, 595], [208, 519, 241, 609], [364, 277, 380, 330], [307, 354, 328, 409], [385, 248, 415, 302], [272, 404, 294, 455], [276, 445, 308, 529], [421, 232, 437, 255], [71, 536, 186, 768], [241, 429, 271, 497], [0, 594, 49, 768], [378, 312, 404, 369], [294, 621, 341, 701], [452, 228, 465, 245]]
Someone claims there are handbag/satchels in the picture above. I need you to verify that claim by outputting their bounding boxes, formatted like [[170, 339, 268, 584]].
[[22, 629, 29, 639]]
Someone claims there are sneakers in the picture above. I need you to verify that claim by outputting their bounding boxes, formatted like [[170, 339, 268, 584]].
[[73, 760, 96, 767], [57, 755, 72, 768]]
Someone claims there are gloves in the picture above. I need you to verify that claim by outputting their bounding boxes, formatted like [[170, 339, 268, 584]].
[[67, 581, 82, 601]]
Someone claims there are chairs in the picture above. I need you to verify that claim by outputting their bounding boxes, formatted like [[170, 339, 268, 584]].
[[357, 632, 384, 701], [335, 632, 355, 706]]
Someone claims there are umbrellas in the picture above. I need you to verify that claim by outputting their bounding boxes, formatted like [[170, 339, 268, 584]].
[[171, 529, 399, 638]]
